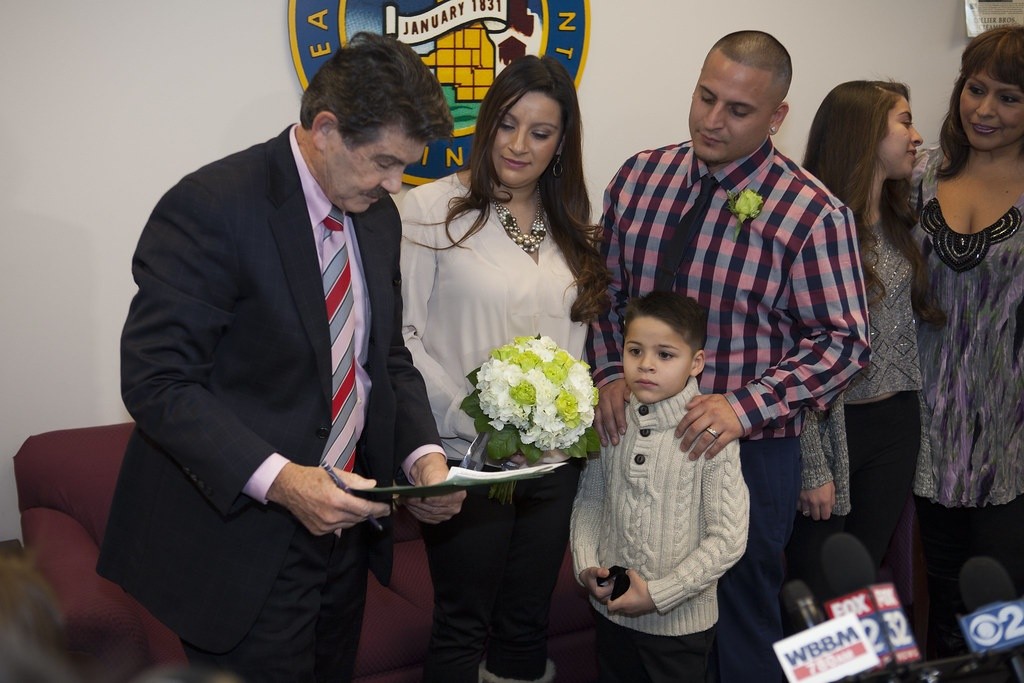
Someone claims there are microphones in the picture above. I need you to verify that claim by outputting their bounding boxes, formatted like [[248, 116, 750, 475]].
[[823, 533, 922, 683], [772, 581, 881, 683], [959, 556, 1024, 683]]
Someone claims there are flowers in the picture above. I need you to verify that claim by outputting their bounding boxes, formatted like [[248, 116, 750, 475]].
[[463, 337, 598, 461]]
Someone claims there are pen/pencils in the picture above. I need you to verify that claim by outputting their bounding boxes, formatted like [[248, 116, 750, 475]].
[[321, 461, 383, 531]]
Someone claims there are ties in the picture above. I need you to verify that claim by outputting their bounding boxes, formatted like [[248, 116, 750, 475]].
[[319, 204, 357, 493], [653, 175, 721, 293]]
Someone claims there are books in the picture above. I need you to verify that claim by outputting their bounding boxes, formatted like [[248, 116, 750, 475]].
[[348, 463, 568, 502]]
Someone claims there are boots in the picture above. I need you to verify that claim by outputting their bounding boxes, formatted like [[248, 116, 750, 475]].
[[478, 658, 556, 683]]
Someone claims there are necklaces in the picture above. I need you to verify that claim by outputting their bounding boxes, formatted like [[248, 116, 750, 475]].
[[486, 182, 546, 253]]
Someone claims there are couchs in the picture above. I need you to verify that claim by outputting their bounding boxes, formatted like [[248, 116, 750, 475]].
[[14, 423, 929, 683]]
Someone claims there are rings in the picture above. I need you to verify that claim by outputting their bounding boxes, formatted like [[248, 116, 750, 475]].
[[706, 428, 719, 438]]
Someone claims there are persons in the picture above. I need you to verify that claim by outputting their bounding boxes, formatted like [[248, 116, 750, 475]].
[[400, 55, 589, 683], [779, 26, 1024, 661], [95, 33, 466, 683], [570, 29, 871, 683]]
